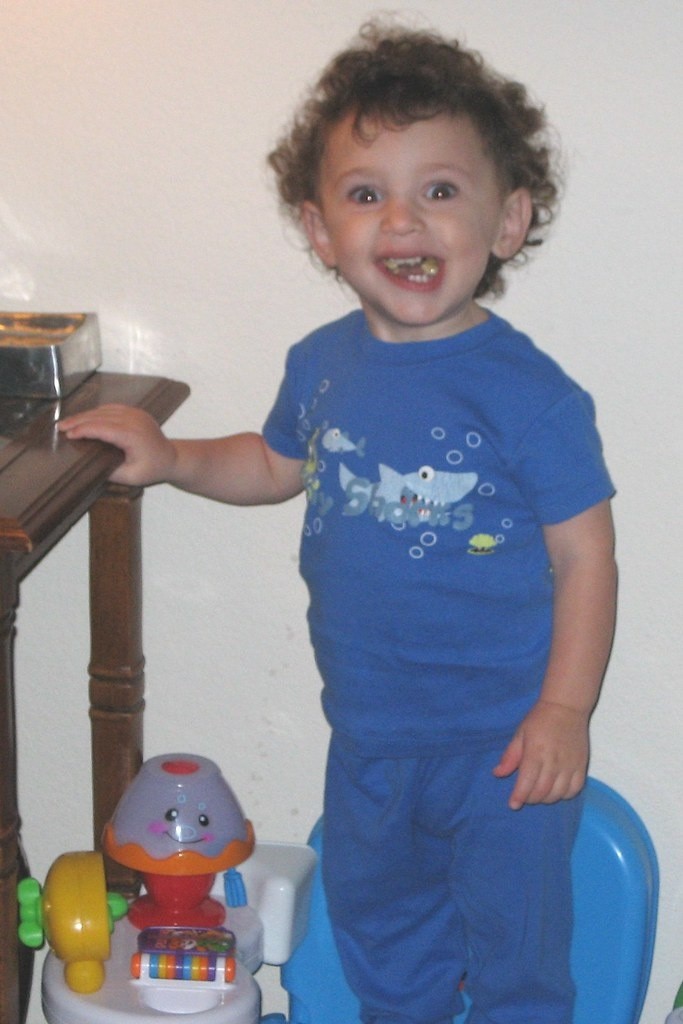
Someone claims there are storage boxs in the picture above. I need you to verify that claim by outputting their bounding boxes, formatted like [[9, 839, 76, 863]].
[[0, 311, 103, 400]]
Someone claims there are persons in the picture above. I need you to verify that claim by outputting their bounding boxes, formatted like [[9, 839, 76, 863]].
[[58, 23, 619, 1024]]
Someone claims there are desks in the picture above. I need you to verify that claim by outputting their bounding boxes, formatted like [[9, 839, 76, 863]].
[[0, 372, 191, 1024]]
